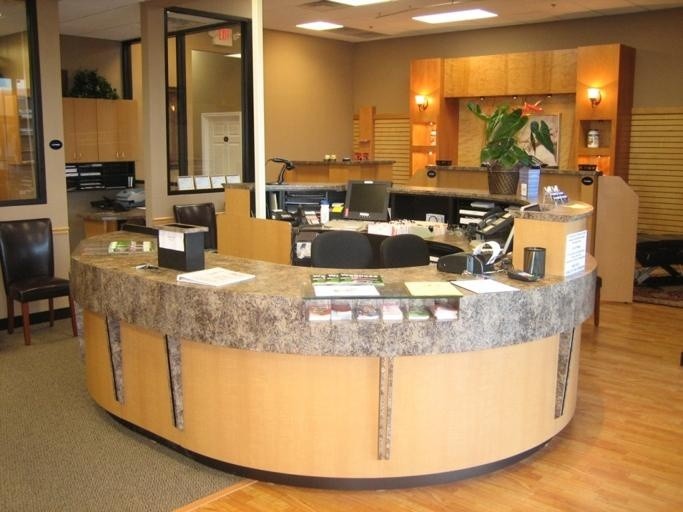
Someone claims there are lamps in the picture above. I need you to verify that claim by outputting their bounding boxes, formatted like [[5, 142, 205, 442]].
[[587, 88, 601, 108], [415, 96, 425, 112]]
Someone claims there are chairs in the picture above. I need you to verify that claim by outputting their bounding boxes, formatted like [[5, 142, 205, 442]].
[[378, 235, 430, 268], [0, 218, 77, 345], [173, 202, 216, 248], [312, 230, 374, 266]]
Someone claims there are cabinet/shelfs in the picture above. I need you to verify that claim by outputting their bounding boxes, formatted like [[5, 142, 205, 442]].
[[4, 96, 35, 166], [62, 97, 138, 164]]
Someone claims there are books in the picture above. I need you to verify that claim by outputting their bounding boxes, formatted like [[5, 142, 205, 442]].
[[176, 266, 255, 286], [457, 207, 490, 216], [106, 239, 157, 254], [469, 201, 494, 208], [450, 278, 520, 294], [460, 216, 484, 225], [321, 220, 368, 232], [310, 273, 384, 286], [404, 280, 463, 298]]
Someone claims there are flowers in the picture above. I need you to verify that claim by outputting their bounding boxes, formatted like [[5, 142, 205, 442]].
[[466, 100, 559, 171]]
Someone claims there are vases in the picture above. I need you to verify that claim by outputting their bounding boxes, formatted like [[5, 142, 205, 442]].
[[486, 167, 521, 196]]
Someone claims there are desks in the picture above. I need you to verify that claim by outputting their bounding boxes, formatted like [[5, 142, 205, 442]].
[[285, 160, 397, 183], [77, 206, 145, 238], [71, 211, 598, 491]]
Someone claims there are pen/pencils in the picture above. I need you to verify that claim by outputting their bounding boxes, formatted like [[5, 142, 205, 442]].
[[529, 248, 536, 274]]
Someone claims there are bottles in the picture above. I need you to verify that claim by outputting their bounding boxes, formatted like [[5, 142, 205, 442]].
[[587, 128, 600, 148], [319, 200, 330, 225]]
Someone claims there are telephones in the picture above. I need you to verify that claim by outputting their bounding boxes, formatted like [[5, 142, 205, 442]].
[[474, 211, 513, 236], [297, 208, 320, 225]]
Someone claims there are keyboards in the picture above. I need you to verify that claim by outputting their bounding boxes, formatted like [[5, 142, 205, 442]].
[[322, 217, 367, 232]]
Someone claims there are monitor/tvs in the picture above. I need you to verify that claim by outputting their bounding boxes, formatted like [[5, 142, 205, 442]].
[[343, 180, 392, 221]]
